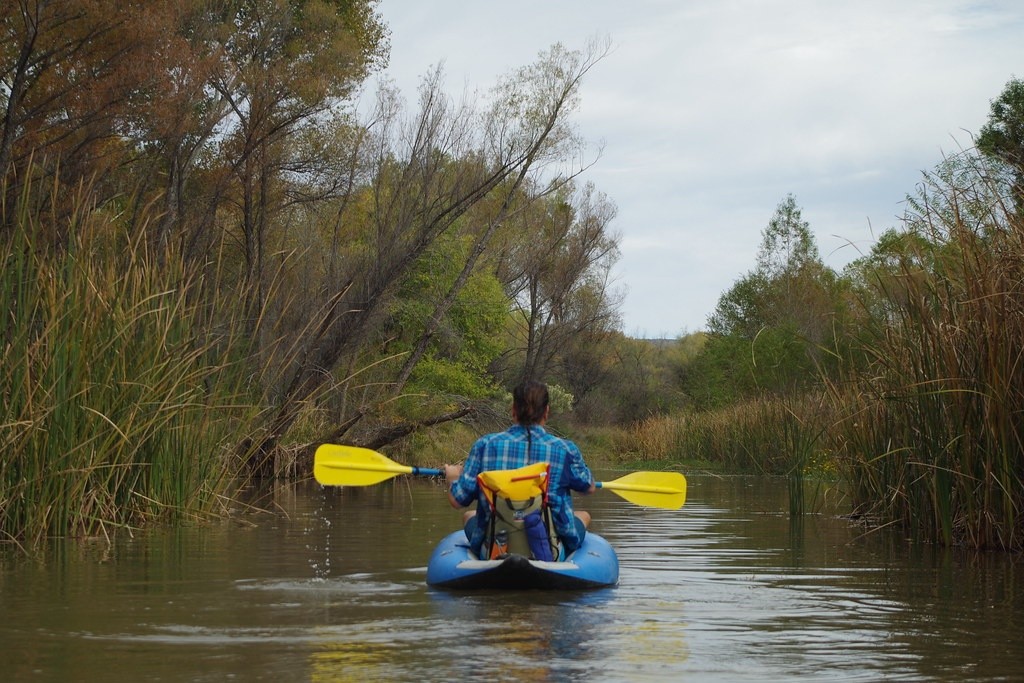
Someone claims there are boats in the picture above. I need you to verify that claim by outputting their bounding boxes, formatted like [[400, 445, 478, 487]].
[[426, 530, 619, 591]]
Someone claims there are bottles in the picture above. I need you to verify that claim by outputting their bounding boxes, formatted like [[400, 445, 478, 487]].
[[491, 530, 508, 558]]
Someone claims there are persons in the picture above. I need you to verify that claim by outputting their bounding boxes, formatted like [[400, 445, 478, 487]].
[[446, 380, 596, 564]]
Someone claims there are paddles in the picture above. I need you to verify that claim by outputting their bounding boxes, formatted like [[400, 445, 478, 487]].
[[313, 444, 686, 514]]
